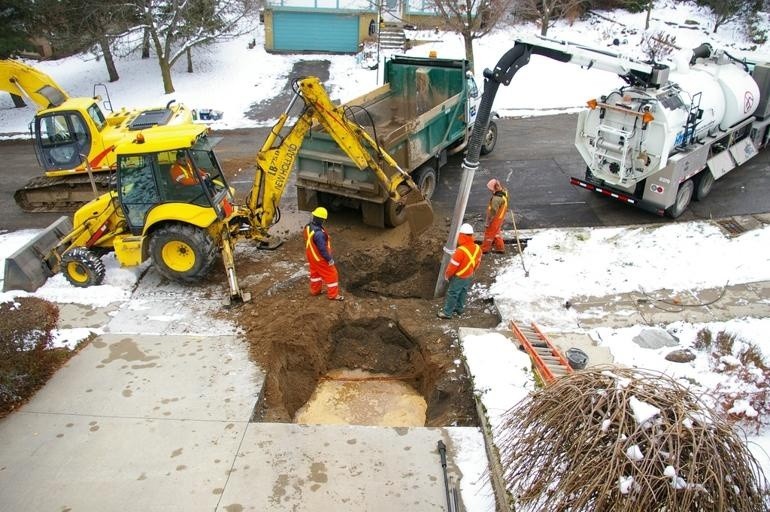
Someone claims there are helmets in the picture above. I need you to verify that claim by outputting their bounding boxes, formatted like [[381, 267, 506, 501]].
[[312, 207, 328, 220], [460, 223, 474, 235]]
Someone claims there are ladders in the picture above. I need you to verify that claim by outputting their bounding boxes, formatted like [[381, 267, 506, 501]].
[[510, 319, 575, 385]]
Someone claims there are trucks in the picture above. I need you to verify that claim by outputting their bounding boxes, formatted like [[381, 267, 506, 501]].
[[294, 50, 500, 228]]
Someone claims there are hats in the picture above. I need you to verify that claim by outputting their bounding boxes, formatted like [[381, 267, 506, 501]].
[[487, 179, 502, 191]]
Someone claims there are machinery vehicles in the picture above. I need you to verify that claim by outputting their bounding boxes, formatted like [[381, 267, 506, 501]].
[[3, 78, 434, 308], [0, 57, 212, 211], [433, 34, 769, 300]]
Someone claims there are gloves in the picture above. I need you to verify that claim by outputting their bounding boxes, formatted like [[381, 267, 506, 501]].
[[328, 258, 335, 266]]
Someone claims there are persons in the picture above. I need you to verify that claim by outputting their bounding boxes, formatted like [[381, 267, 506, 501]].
[[301, 205, 346, 302], [435, 222, 483, 320], [478, 177, 510, 254], [169, 152, 214, 202]]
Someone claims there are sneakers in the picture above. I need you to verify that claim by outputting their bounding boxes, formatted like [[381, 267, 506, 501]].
[[492, 250, 505, 253], [335, 294, 344, 301], [437, 310, 452, 319]]
